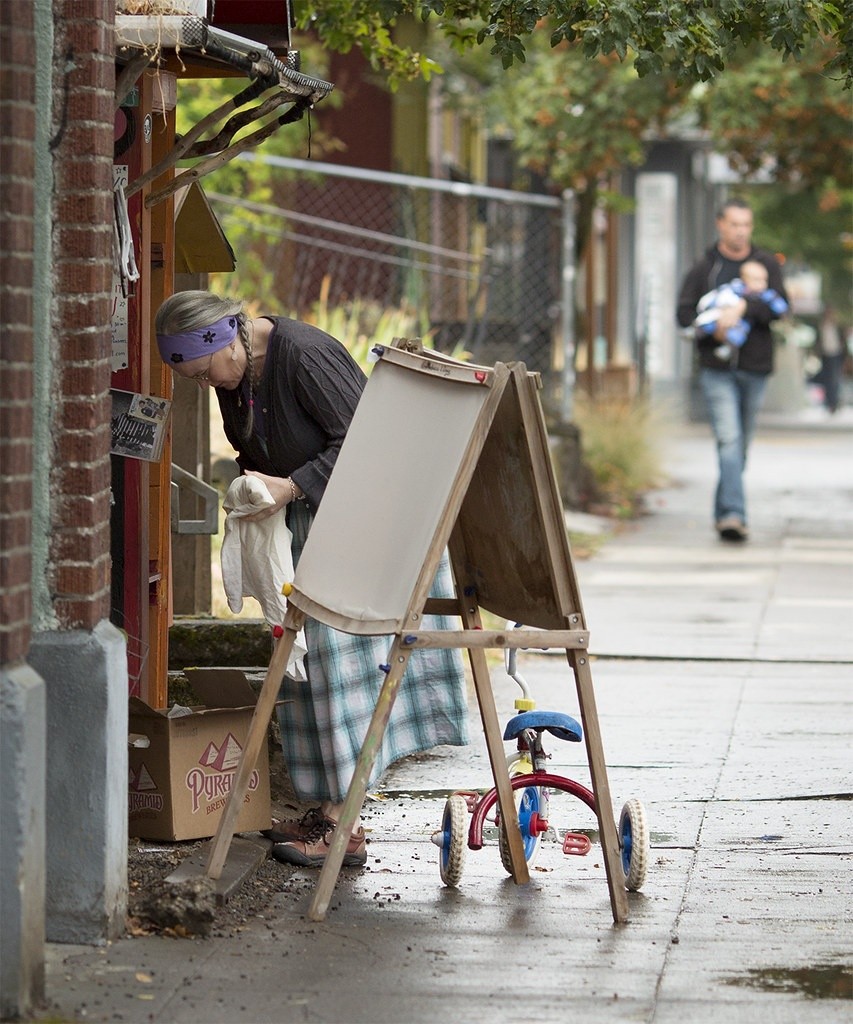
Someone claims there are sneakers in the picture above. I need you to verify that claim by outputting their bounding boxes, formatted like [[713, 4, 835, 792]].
[[714, 512, 750, 541], [259, 807, 329, 844], [271, 816, 369, 869]]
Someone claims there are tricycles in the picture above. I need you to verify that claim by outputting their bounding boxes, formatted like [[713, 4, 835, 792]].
[[431, 618, 651, 892]]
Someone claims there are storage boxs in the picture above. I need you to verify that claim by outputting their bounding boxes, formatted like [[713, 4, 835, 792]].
[[127, 665, 294, 843]]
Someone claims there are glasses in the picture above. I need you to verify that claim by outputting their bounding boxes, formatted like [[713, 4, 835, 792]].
[[184, 353, 212, 383]]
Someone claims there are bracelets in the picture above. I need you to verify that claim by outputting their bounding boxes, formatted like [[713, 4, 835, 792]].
[[288, 476, 296, 503]]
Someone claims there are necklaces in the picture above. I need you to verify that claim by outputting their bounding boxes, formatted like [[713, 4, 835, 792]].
[[237, 320, 254, 407]]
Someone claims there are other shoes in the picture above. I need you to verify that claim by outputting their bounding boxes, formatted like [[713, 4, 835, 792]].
[[678, 327, 697, 340], [712, 344, 732, 361]]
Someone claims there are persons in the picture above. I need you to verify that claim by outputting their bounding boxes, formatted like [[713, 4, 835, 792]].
[[679, 262, 789, 363], [155, 290, 469, 867], [677, 199, 788, 541], [816, 312, 848, 415]]
